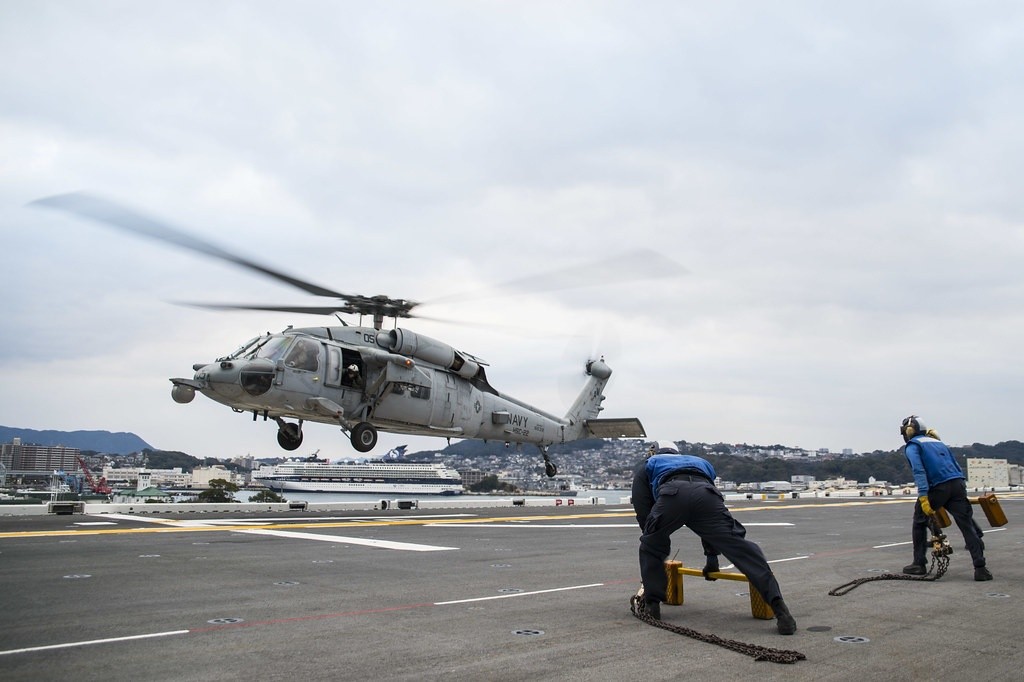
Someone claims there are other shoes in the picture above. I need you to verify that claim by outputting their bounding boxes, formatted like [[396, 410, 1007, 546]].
[[903, 565, 926, 575], [975, 567, 993, 580]]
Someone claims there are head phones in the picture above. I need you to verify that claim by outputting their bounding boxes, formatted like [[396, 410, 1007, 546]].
[[905, 417, 915, 437]]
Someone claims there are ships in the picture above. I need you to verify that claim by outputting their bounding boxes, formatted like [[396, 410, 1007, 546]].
[[252, 461, 466, 495]]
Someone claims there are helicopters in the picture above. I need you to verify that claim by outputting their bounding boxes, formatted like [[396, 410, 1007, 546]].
[[29, 192, 648, 478]]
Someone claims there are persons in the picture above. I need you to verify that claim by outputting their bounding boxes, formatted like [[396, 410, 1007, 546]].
[[900, 414, 994, 581], [341, 364, 363, 388], [630, 438, 798, 636], [286, 338, 318, 369]]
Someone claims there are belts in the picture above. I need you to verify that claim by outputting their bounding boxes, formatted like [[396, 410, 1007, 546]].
[[675, 476, 707, 482]]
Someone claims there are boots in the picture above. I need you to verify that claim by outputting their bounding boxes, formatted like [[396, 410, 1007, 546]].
[[771, 601, 796, 635], [644, 602, 660, 619]]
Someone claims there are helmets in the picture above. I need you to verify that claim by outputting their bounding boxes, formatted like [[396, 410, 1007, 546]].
[[901, 416, 927, 443], [653, 440, 679, 454], [348, 364, 359, 371]]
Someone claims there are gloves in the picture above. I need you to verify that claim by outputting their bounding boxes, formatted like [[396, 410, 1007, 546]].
[[702, 555, 719, 582], [919, 496, 933, 516], [356, 371, 360, 379]]
